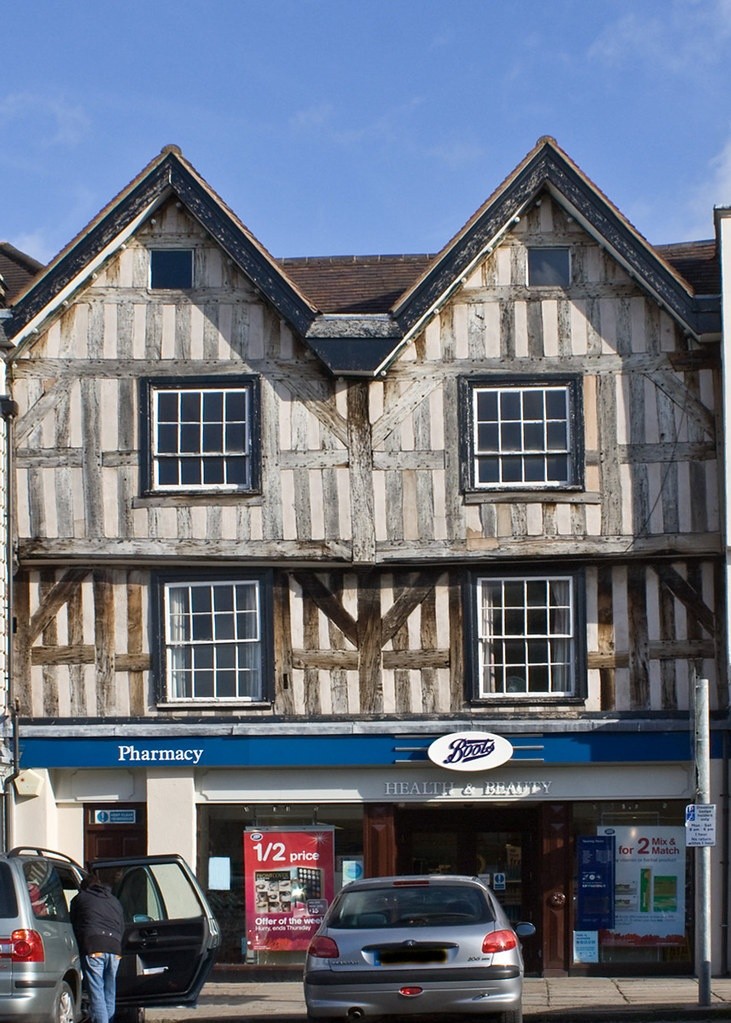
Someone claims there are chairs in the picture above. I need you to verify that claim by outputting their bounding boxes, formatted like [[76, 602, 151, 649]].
[[445, 900, 477, 915]]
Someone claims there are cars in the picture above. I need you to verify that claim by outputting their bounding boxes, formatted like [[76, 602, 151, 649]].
[[302, 873, 536, 1023]]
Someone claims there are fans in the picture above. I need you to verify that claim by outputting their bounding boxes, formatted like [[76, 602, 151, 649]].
[[499, 676, 525, 693]]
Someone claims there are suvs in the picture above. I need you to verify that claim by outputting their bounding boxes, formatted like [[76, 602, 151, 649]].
[[0, 846, 223, 1023]]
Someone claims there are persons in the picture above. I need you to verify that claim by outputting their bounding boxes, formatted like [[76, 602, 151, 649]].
[[69, 874, 127, 1023]]
